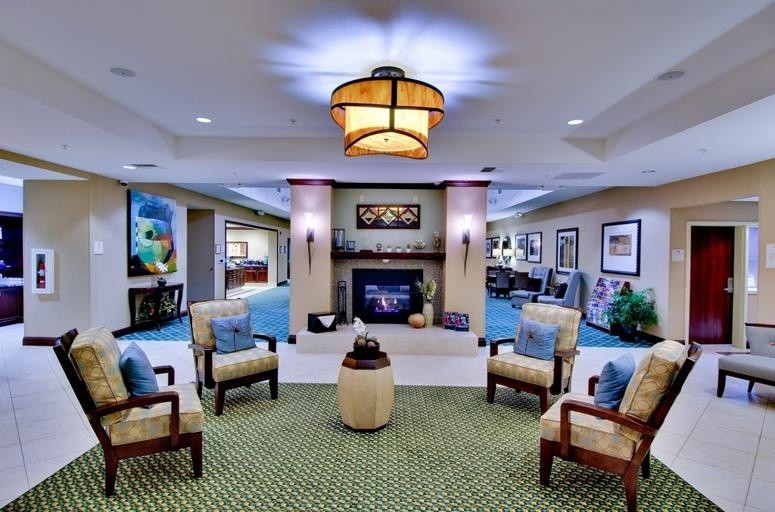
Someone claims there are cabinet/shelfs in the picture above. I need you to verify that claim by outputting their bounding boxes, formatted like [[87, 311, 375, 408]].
[[245, 266, 267, 283]]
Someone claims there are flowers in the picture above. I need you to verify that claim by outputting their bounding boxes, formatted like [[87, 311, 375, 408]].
[[353, 317, 369, 341]]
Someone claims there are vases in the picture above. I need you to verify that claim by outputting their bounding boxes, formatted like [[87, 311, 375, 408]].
[[353, 340, 380, 359]]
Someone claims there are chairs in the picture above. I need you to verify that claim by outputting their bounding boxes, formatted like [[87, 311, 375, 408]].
[[187, 299, 279, 415], [487, 266, 580, 309], [52, 326, 204, 496], [485, 301, 583, 414], [540, 339, 702, 511]]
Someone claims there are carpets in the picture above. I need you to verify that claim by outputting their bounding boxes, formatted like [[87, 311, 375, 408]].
[[485, 287, 661, 348], [116, 284, 289, 341], [0, 382, 724, 511]]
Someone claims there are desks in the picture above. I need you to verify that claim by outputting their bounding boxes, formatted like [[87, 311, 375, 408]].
[[130, 283, 184, 331]]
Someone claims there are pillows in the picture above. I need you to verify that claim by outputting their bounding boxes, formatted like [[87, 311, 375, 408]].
[[594, 353, 635, 420], [212, 314, 255, 353], [119, 340, 157, 409], [514, 319, 560, 360]]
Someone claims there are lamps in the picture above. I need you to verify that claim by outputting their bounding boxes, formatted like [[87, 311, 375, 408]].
[[462, 214, 473, 244], [302, 213, 315, 242], [330, 67, 446, 160]]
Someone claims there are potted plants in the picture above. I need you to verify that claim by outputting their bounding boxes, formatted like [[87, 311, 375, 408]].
[[415, 280, 436, 328], [387, 243, 411, 252], [600, 287, 658, 341]]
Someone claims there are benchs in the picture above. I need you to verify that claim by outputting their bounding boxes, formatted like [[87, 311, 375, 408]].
[[717, 323, 774, 399]]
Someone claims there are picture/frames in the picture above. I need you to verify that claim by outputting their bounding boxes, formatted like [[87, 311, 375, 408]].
[[487, 219, 643, 276], [226, 241, 248, 258]]
